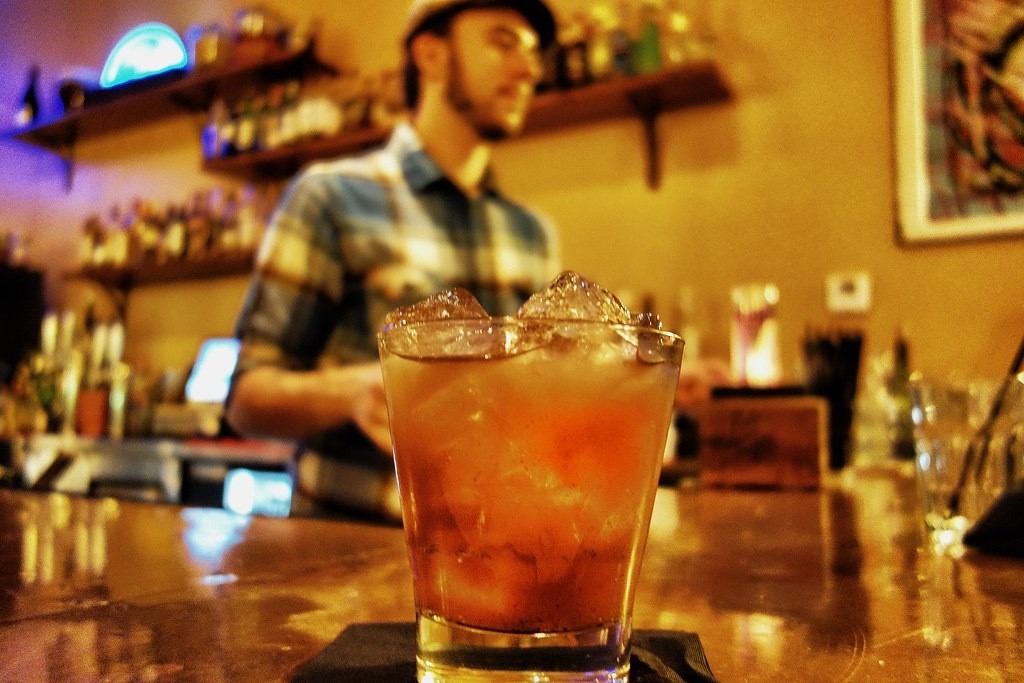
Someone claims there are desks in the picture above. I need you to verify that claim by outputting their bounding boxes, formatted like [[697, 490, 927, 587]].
[[0, 492, 1024, 683]]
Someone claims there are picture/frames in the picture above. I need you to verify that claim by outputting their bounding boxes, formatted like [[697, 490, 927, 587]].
[[890, 0, 1024, 247]]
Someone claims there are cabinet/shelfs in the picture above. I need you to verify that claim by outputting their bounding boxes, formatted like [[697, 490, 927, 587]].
[[2, 40, 738, 320]]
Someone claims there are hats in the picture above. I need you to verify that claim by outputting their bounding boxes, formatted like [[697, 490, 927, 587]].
[[402, 0, 559, 56]]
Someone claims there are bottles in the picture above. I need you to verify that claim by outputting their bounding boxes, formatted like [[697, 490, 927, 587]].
[[24, 308, 149, 439], [196, 11, 697, 165], [69, 186, 271, 273]]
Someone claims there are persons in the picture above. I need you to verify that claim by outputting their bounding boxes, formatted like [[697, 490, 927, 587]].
[[224, 0, 561, 526]]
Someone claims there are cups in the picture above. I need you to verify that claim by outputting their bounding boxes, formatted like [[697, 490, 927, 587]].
[[909, 371, 1024, 551], [375, 316, 685, 683]]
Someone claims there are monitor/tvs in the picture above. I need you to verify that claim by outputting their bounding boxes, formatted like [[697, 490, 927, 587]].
[[182, 335, 243, 405]]
